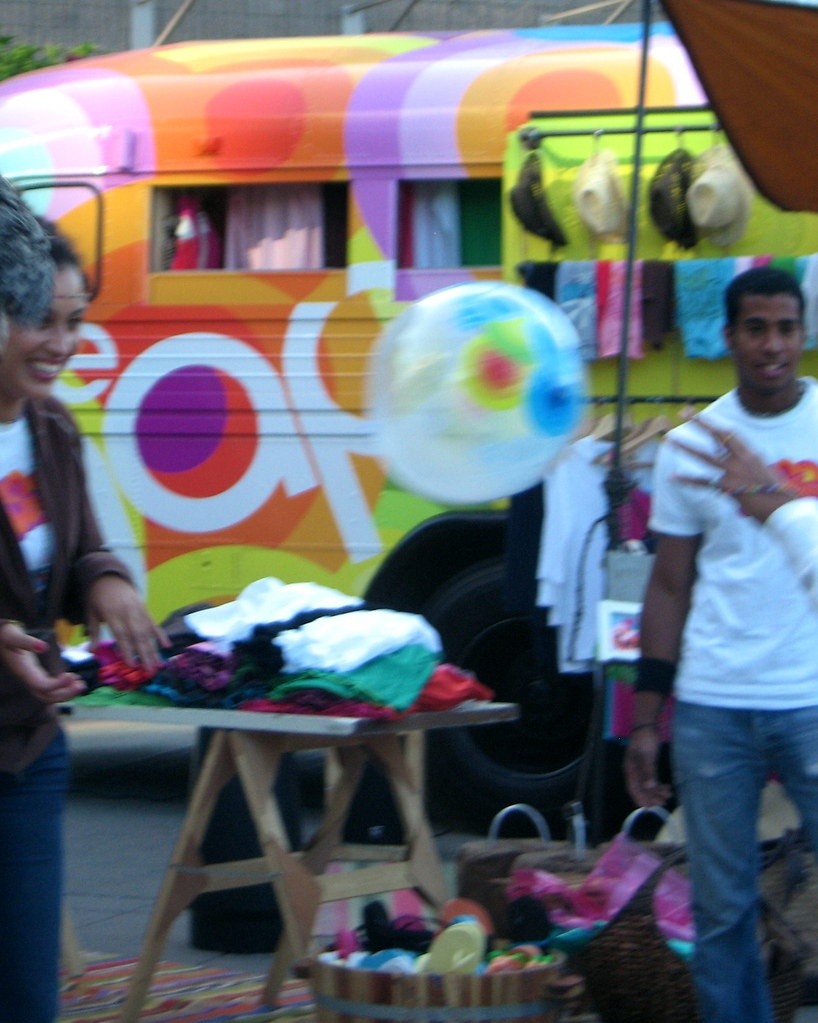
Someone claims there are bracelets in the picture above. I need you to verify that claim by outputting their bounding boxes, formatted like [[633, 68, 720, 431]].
[[633, 722, 656, 731], [634, 657, 677, 696]]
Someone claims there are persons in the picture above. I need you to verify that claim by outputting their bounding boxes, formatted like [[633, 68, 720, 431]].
[[0, 176, 172, 1023], [627, 268, 818, 1023], [658, 407, 818, 605]]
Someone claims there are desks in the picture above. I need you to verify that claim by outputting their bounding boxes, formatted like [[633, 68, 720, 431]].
[[72, 701, 523, 1022]]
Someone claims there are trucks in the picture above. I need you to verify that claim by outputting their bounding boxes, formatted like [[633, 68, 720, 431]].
[[1, 24, 817, 841]]
